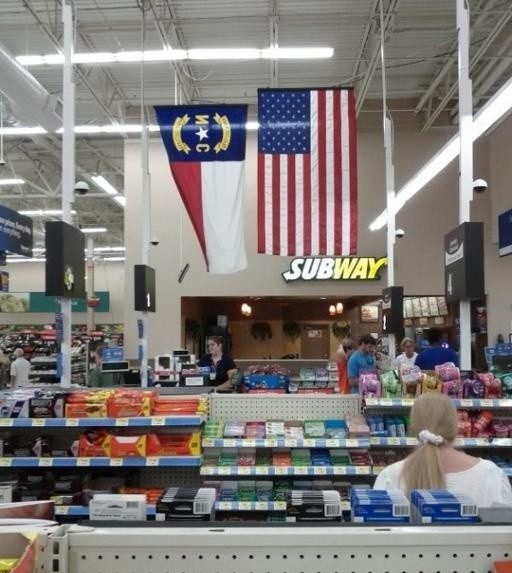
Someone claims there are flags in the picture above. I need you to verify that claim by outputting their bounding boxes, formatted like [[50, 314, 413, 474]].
[[154, 104, 248, 273], [258, 88, 358, 257]]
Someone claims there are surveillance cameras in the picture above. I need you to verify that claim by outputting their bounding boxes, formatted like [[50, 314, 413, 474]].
[[395, 229, 405, 238], [473, 179, 488, 194], [74, 181, 89, 194], [150, 238, 159, 246]]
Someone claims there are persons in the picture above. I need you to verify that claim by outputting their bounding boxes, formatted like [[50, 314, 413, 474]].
[[336, 335, 377, 394], [373, 392, 512, 508], [197, 336, 238, 393], [10, 348, 31, 389], [396, 337, 419, 368], [87, 346, 133, 387]]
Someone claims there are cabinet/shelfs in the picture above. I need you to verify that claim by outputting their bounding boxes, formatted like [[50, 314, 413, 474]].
[[0, 395, 206, 524], [362, 394, 512, 490], [199, 396, 372, 525]]
